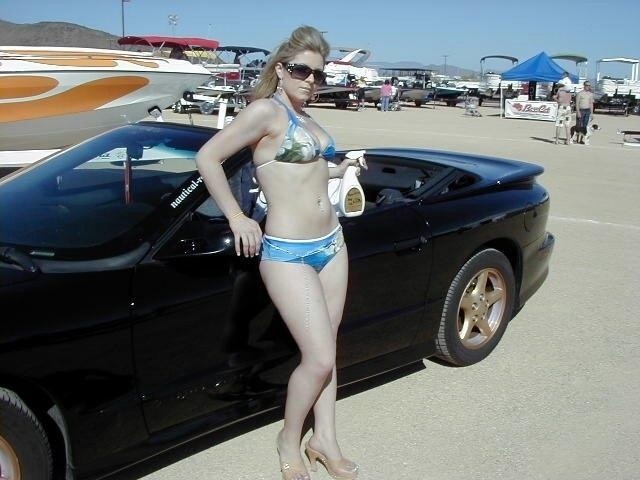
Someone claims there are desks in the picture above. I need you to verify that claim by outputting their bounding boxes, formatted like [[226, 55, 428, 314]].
[[505, 98, 559, 122]]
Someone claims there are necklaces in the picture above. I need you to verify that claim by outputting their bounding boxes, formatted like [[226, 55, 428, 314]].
[[295, 113, 306, 123]]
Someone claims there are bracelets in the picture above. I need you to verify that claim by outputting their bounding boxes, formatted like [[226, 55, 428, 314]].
[[229, 212, 244, 220]]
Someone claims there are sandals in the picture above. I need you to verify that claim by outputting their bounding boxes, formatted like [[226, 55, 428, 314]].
[[277, 439, 311, 480]]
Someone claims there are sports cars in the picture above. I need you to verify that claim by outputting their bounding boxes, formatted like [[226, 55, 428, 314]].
[[0, 119, 554, 479]]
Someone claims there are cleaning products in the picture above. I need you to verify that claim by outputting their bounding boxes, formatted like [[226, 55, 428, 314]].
[[339, 148, 367, 218]]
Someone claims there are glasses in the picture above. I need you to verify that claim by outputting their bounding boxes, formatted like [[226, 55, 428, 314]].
[[281, 61, 328, 86]]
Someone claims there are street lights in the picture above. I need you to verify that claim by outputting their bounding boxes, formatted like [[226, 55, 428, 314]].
[[121, 0, 131, 37], [168, 12, 180, 35]]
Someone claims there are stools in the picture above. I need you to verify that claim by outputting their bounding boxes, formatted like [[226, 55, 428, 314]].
[[621, 130, 640, 143]]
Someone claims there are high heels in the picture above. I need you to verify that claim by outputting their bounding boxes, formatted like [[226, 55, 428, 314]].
[[304, 438, 360, 480]]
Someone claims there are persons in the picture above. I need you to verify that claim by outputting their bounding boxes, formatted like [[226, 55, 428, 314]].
[[553, 72, 572, 99], [194, 23, 361, 480], [575, 81, 593, 144], [467, 98, 477, 113], [380, 79, 393, 112], [357, 76, 366, 108], [552, 84, 570, 145]]
